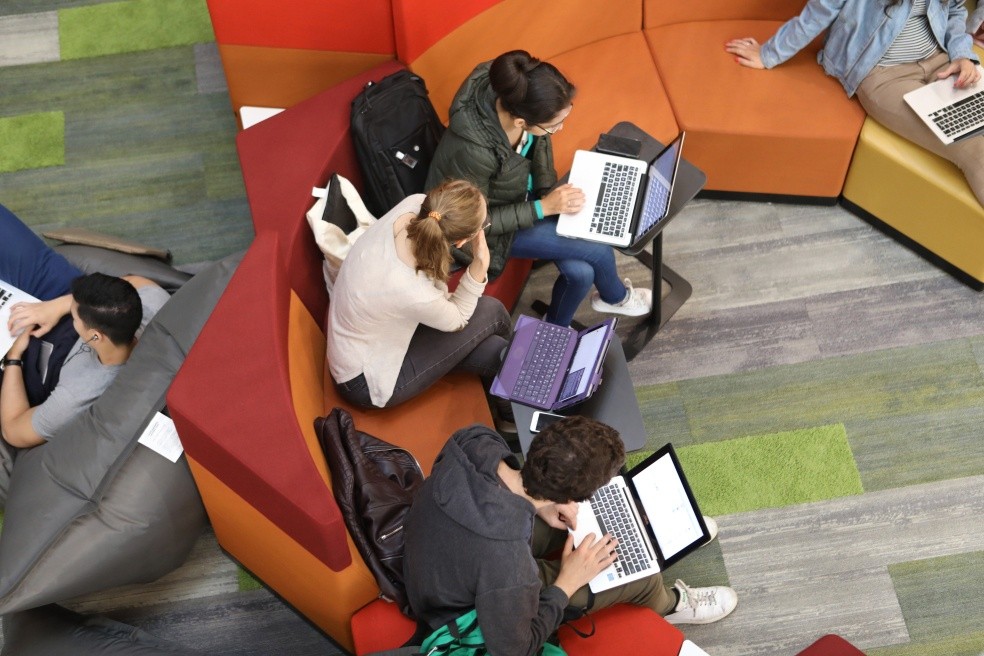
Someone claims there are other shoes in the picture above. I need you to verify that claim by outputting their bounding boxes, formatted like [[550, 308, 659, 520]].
[[495, 416, 519, 441]]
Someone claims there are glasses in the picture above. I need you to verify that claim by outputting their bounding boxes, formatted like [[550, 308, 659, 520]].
[[465, 214, 492, 236], [509, 111, 561, 137]]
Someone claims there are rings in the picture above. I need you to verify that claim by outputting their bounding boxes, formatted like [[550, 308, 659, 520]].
[[744, 42, 751, 46], [568, 200, 571, 207]]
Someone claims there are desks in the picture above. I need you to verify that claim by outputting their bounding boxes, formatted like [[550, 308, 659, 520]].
[[544, 121, 705, 366]]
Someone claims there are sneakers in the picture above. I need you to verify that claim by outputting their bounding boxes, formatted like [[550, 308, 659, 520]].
[[663, 579, 738, 624], [541, 313, 574, 329], [700, 516, 718, 548], [590, 277, 652, 316]]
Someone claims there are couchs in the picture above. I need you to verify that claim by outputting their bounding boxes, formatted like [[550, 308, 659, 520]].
[[164, 0, 984, 656], [0, 247, 232, 613]]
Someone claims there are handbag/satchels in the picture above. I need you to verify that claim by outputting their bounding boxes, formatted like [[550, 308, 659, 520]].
[[418, 609, 568, 656], [305, 172, 378, 295]]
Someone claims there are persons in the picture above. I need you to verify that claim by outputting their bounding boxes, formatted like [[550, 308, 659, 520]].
[[326, 176, 517, 433], [403, 416, 739, 656], [725, 0, 984, 209], [425, 49, 652, 329], [0, 203, 172, 446]]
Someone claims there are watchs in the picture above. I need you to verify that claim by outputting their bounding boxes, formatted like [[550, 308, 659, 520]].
[[0, 360, 23, 370]]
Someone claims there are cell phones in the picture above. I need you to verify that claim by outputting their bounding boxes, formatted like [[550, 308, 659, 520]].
[[597, 134, 641, 158], [529, 411, 566, 433]]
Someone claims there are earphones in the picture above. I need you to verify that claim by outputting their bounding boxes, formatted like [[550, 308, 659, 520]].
[[92, 335, 98, 340]]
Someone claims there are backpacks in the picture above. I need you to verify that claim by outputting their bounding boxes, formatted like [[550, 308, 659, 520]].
[[350, 70, 449, 220]]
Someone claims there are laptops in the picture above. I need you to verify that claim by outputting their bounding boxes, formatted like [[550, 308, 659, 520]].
[[489, 314, 620, 413], [903, 64, 984, 146], [555, 131, 685, 249], [568, 443, 711, 593], [0, 279, 43, 360]]
